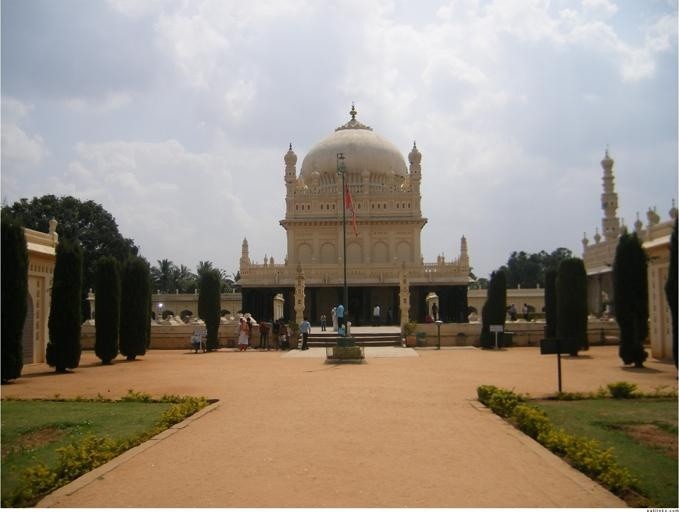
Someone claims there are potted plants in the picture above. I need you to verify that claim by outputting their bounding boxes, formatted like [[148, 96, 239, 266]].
[[287, 320, 299, 349], [399, 319, 418, 346]]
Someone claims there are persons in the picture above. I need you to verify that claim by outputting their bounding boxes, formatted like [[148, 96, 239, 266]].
[[431, 302, 438, 323], [523, 304, 529, 320], [602, 291, 610, 315], [299, 317, 310, 350], [238, 317, 288, 352], [511, 303, 517, 321], [320, 313, 326, 331], [192, 326, 207, 353], [373, 304, 381, 326], [331, 303, 345, 332], [387, 307, 393, 322]]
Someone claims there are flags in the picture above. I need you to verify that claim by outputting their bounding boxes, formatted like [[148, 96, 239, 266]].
[[345, 183, 357, 234]]
